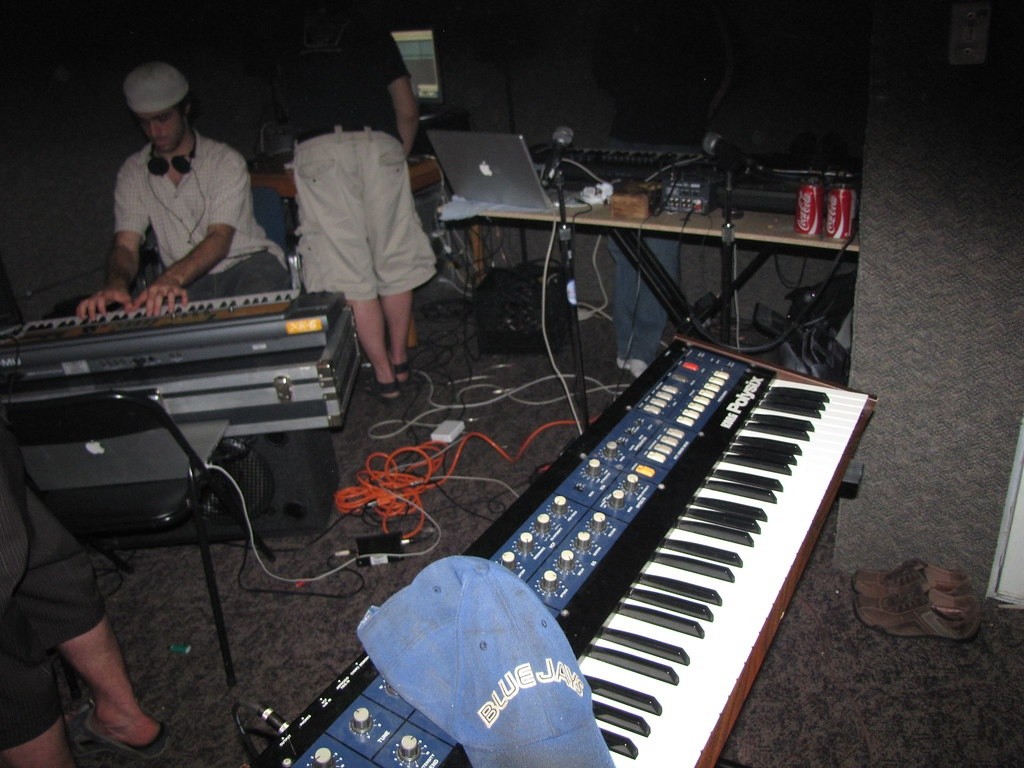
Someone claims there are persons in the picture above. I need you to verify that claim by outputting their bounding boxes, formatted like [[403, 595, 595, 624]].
[[0, 406, 167, 768], [284, 1, 438, 397], [75, 61, 289, 320]]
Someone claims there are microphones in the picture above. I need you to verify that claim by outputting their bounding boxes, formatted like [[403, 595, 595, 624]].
[[541, 125, 574, 187], [703, 131, 753, 165]]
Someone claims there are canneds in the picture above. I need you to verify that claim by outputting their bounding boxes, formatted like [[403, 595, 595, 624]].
[[824, 184, 857, 240], [794, 182, 824, 236]]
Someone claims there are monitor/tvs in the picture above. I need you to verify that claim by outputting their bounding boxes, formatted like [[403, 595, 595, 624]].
[[388, 22, 445, 104]]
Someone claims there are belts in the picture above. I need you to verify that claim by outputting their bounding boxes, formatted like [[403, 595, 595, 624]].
[[296, 126, 381, 144]]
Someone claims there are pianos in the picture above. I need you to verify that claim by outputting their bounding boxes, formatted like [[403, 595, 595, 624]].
[[0, 288, 347, 386], [242, 333, 880, 768]]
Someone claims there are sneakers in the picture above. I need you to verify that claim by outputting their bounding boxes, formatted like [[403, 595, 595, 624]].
[[853, 558, 972, 596], [854, 584, 982, 641]]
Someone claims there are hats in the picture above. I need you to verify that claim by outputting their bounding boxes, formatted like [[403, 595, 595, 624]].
[[120, 62, 189, 113], [357, 555, 616, 768]]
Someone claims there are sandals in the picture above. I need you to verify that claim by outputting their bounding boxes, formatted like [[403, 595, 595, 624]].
[[392, 363, 412, 387], [371, 375, 402, 404]]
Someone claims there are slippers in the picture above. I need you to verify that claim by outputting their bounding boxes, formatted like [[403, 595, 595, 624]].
[[62, 704, 169, 759]]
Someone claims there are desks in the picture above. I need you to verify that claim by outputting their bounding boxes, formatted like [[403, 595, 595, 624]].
[[252, 154, 485, 349], [437, 186, 860, 349]]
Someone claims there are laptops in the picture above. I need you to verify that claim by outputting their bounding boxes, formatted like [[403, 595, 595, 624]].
[[21, 395, 229, 490], [426, 129, 576, 210]]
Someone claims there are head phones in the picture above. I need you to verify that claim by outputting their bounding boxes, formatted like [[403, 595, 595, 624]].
[[147, 130, 196, 175]]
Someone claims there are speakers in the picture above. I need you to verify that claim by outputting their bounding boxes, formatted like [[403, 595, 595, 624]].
[[74, 425, 343, 550]]
